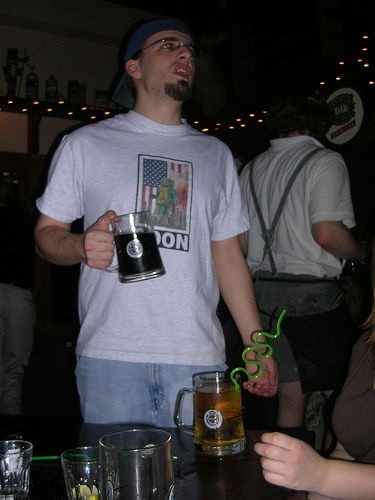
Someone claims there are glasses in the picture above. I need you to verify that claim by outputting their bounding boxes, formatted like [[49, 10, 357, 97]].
[[135, 39, 198, 57]]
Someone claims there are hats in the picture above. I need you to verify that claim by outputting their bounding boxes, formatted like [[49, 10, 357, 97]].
[[111, 18, 200, 109]]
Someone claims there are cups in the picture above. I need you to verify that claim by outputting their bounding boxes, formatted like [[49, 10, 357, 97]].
[[60, 446, 100, 500], [104, 210, 166, 283], [0, 440, 33, 500], [99, 428, 176, 500], [174, 370, 248, 459]]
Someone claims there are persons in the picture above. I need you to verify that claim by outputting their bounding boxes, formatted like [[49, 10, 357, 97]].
[[254, 293, 374, 500], [33, 19, 279, 500], [0, 195, 34, 414], [239, 92, 375, 428]]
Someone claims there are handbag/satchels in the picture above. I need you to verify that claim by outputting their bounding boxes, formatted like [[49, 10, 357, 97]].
[[279, 305, 360, 394]]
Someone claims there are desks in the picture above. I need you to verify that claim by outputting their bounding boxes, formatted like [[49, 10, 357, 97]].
[[0, 413, 315, 500]]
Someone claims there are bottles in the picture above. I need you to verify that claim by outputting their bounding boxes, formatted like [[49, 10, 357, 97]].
[[26, 69, 40, 100], [46, 74, 57, 103]]
